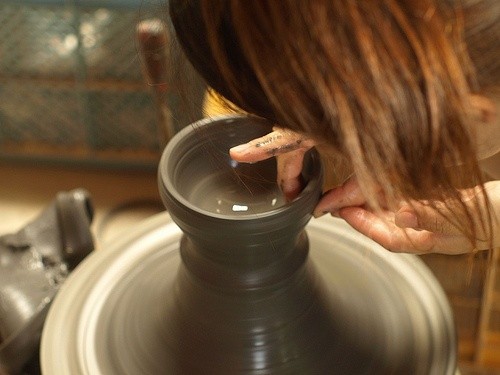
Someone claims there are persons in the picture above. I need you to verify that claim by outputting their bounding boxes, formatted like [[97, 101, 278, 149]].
[[167, 0, 499, 262]]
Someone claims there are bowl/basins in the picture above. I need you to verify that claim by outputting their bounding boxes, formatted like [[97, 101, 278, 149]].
[[159, 112, 324, 252]]
